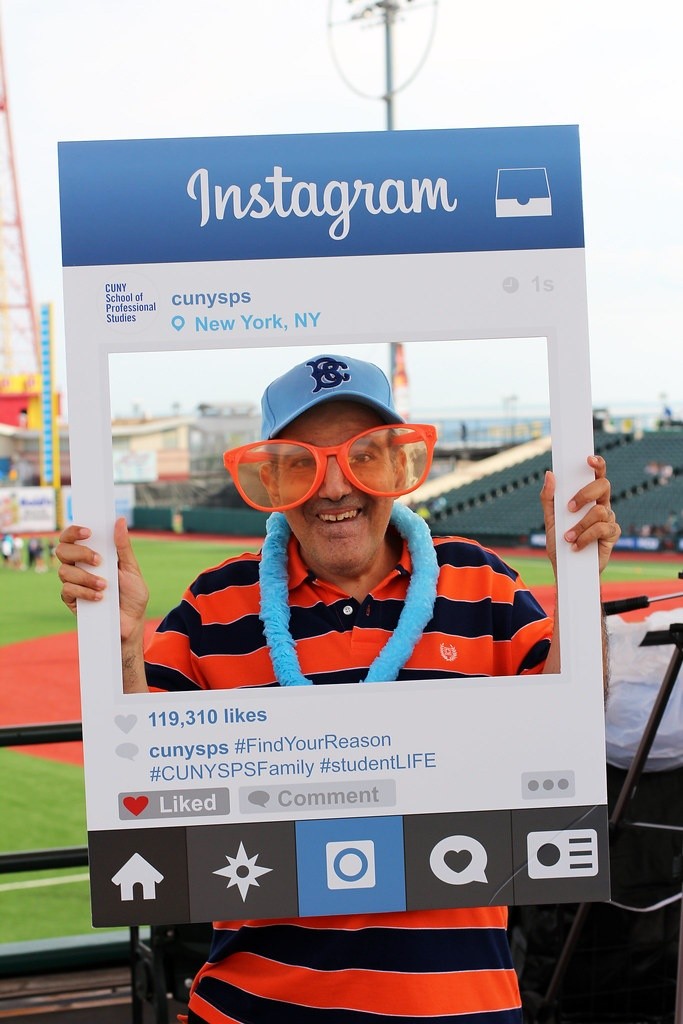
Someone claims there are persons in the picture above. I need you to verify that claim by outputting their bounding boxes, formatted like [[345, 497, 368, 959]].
[[625, 510, 681, 549], [643, 459, 674, 485], [0, 531, 57, 572], [56, 352, 622, 1024]]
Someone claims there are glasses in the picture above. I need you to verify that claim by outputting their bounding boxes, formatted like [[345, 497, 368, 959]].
[[222, 422, 439, 513]]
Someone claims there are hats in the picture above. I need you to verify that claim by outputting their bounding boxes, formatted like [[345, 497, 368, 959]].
[[259, 353, 407, 443]]
